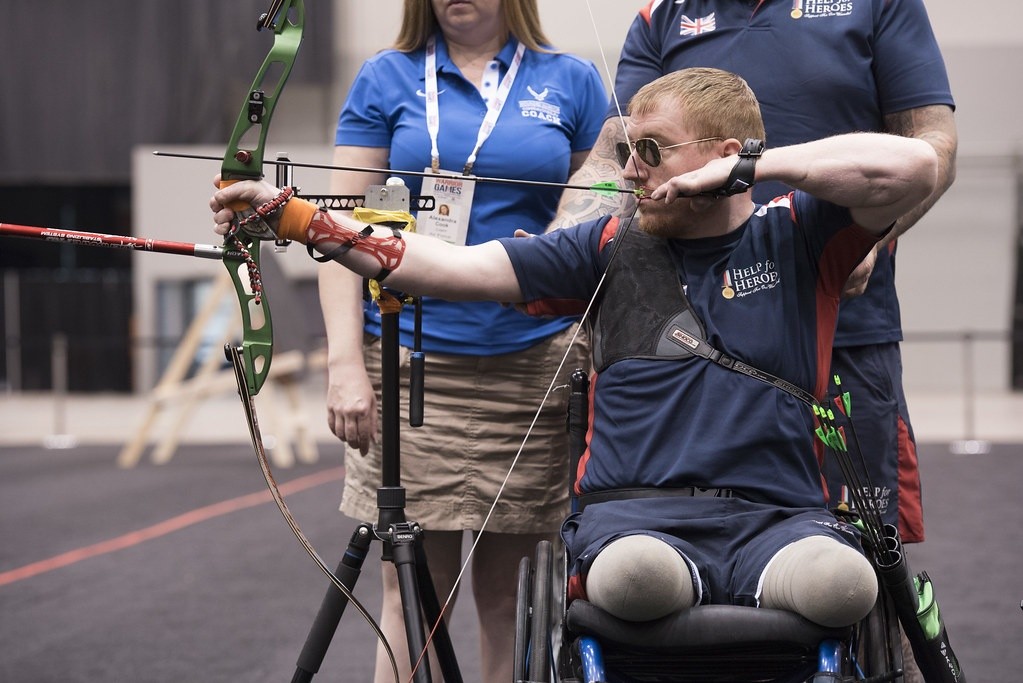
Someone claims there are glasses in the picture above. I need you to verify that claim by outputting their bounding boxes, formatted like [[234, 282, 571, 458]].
[[616, 137, 726, 170]]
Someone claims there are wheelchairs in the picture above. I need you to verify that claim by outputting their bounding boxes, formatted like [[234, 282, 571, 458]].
[[512, 368, 908, 683]]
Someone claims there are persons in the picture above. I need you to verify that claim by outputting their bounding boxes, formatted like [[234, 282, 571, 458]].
[[437, 204, 449, 215], [498, 0, 958, 683], [210, 67, 937, 625], [315, 0, 612, 683]]
